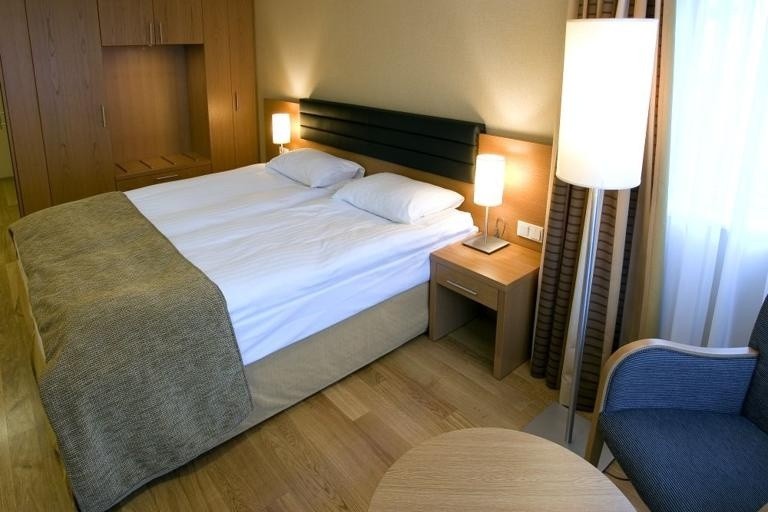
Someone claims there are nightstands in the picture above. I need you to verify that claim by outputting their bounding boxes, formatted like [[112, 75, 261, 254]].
[[426, 234, 542, 380]]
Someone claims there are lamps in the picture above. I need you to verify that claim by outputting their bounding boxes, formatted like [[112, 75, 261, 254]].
[[462, 154, 509, 254], [271, 112, 291, 155], [522, 16, 663, 475]]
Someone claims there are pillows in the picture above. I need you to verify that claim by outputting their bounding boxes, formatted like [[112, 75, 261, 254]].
[[265, 147, 466, 224]]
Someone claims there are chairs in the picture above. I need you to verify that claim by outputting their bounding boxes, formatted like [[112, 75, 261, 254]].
[[583, 294, 768, 511]]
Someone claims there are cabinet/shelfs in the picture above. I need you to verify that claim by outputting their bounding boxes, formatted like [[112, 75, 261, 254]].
[[0, 0, 261, 219]]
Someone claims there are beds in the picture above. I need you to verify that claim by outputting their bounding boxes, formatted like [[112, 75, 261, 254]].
[[7, 95, 555, 508]]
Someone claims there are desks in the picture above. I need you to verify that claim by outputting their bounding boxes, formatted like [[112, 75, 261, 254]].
[[366, 427, 636, 512]]
